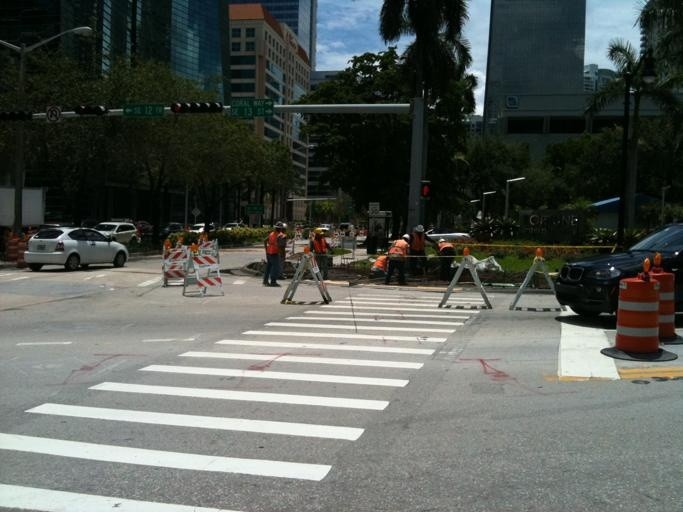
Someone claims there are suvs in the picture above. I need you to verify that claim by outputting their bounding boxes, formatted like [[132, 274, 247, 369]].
[[554, 224, 682, 321]]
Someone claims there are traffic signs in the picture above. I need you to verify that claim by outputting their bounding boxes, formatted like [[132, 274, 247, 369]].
[[122, 104, 164, 117], [230, 100, 274, 118]]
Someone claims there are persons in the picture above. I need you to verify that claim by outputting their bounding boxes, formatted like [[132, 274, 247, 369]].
[[437, 238, 456, 284], [370, 249, 390, 274], [261, 220, 283, 288], [409, 225, 438, 272], [308, 227, 333, 279], [279, 224, 288, 280], [384, 234, 409, 285]]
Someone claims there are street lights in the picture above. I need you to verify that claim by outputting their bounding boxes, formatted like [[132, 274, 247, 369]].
[[610, 45, 658, 255], [0, 24, 93, 238], [470, 177, 525, 239]]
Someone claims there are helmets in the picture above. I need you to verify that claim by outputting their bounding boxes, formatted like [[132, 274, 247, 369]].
[[439, 238, 446, 243], [314, 228, 324, 235], [415, 225, 424, 232], [403, 233, 410, 240], [276, 221, 287, 230]]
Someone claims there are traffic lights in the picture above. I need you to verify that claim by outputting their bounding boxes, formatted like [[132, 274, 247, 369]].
[[170, 103, 223, 113], [420, 180, 431, 198], [75, 105, 108, 115], [0, 111, 31, 121]]
